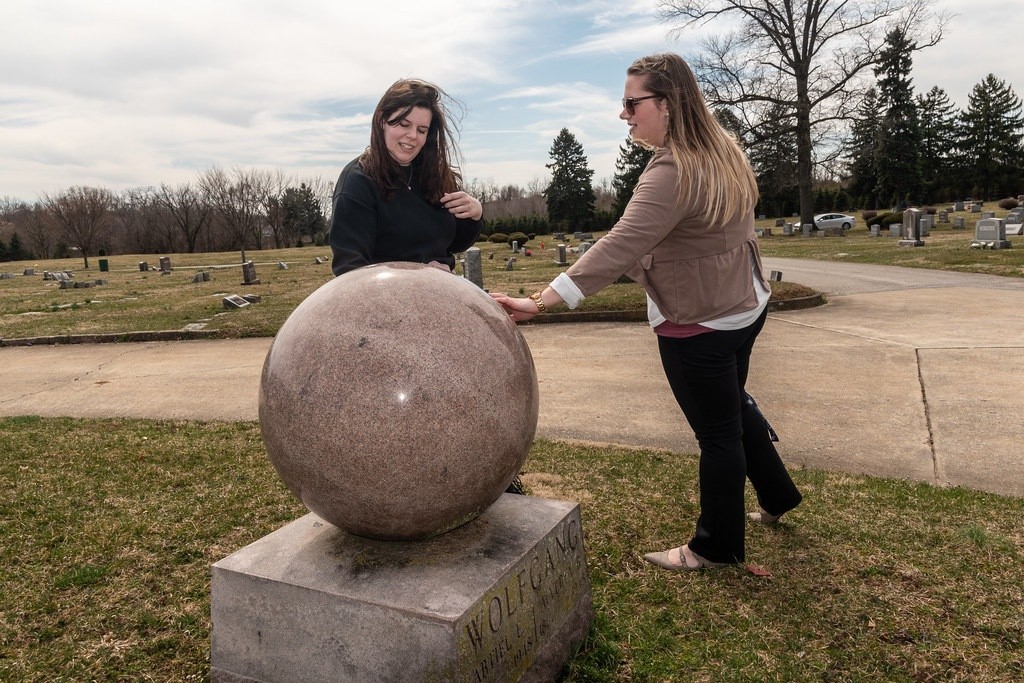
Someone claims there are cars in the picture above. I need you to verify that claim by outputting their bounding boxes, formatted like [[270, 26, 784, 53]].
[[792, 213, 856, 232]]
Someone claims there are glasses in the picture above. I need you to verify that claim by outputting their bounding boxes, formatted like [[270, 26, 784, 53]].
[[622, 93, 666, 116]]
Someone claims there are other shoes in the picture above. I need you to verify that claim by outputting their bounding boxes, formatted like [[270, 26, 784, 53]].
[[746, 502, 783, 523], [642, 544, 726, 570]]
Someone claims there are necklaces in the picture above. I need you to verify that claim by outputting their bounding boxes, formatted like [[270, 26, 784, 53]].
[[396, 164, 413, 191]]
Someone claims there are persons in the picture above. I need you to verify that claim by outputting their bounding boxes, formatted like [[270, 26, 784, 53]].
[[488, 51, 804, 573], [329, 77, 486, 276]]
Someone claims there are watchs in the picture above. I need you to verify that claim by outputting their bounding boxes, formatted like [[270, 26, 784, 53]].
[[530, 291, 549, 314]]
